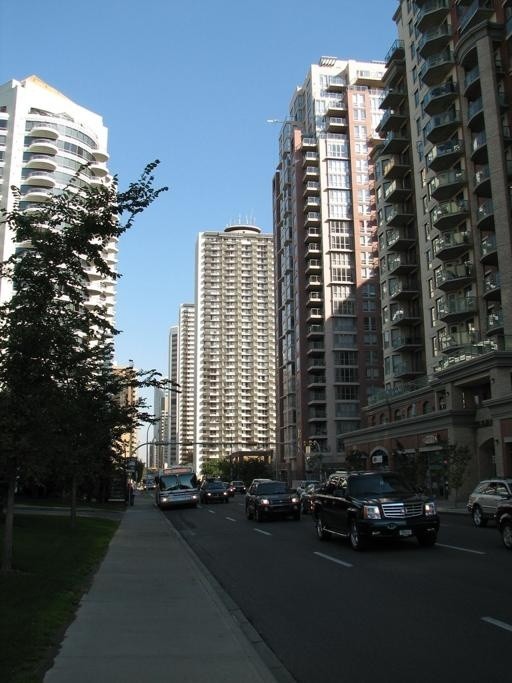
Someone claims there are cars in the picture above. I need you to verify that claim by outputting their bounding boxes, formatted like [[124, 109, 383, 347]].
[[496, 497, 511, 547], [142, 464, 320, 523]]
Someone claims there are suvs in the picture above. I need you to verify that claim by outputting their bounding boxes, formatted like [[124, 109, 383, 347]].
[[310, 469, 440, 548], [467, 478, 511, 528]]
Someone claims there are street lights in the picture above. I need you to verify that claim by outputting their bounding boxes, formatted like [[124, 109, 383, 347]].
[[304, 440, 322, 478], [146, 414, 176, 467]]
